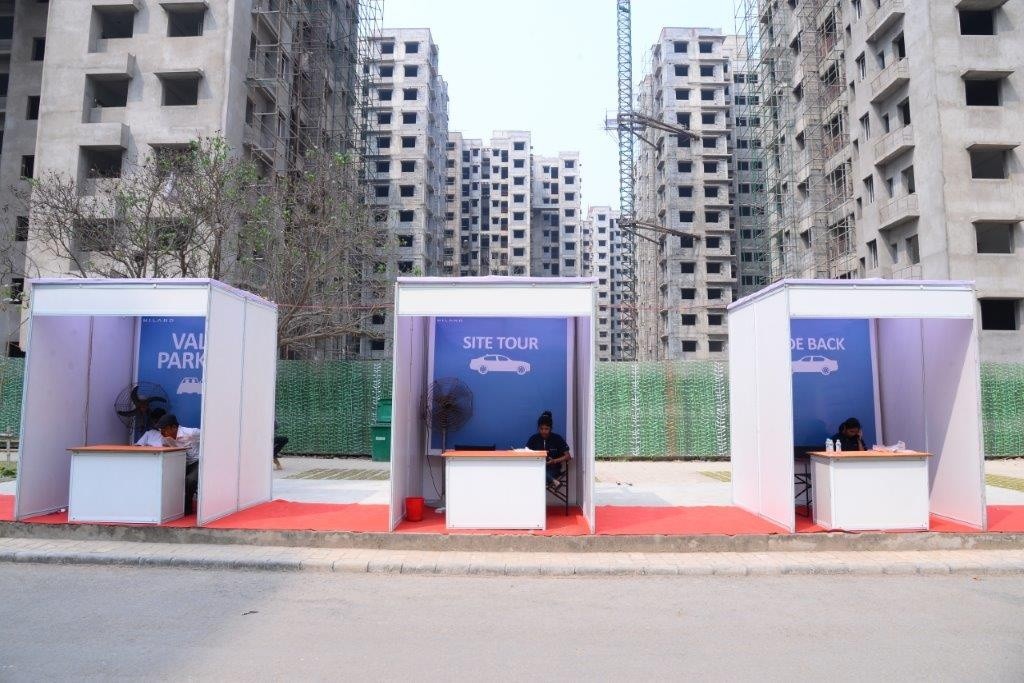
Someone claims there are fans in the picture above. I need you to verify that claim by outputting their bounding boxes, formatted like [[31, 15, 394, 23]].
[[114, 381, 171, 433], [419, 377, 474, 513]]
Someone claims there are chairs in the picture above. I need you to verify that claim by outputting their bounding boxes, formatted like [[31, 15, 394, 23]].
[[794, 473, 812, 519], [547, 460, 569, 516]]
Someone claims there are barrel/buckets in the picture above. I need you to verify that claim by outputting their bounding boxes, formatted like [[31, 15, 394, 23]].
[[406, 497, 424, 521]]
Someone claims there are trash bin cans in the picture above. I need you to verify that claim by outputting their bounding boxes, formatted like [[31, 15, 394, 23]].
[[405, 497, 424, 522], [367, 398, 393, 461]]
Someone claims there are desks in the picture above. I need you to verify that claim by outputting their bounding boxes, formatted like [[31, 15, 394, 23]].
[[441, 451, 547, 530], [806, 450, 933, 532], [65, 445, 193, 524]]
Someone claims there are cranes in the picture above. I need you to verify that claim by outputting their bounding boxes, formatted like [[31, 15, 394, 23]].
[[617, 0, 637, 361]]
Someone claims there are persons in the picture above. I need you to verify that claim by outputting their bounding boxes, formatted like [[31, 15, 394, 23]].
[[524, 411, 572, 489], [830, 417, 869, 451], [135, 415, 201, 517]]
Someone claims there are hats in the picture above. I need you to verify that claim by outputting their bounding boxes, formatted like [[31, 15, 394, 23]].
[[538, 410, 553, 425], [156, 415, 179, 428]]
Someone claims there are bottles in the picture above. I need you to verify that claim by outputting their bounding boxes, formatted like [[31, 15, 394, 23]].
[[825, 438, 834, 451], [836, 439, 841, 452]]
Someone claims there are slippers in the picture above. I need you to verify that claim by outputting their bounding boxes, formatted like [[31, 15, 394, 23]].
[[555, 482, 563, 490]]
